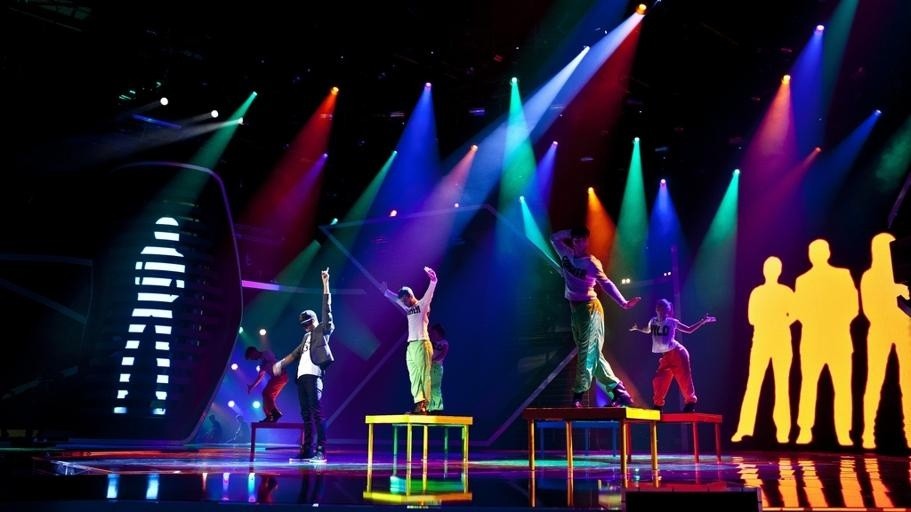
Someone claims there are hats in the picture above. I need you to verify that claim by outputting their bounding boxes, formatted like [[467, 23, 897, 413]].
[[297, 310, 316, 328]]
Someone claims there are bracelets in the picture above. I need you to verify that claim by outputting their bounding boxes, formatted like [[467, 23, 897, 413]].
[[281, 359, 287, 368]]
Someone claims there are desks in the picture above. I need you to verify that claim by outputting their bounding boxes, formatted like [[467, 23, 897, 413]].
[[251, 421, 306, 461]]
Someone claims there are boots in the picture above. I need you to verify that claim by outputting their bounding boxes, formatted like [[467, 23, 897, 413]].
[[289, 445, 327, 463], [259, 409, 283, 423], [573, 391, 584, 408], [653, 405, 663, 413], [683, 403, 696, 413], [604, 381, 634, 407]]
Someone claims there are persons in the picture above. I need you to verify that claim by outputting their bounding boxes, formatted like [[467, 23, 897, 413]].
[[231, 414, 250, 444], [862, 452, 911, 511], [548, 223, 642, 408], [427, 325, 449, 414], [376, 266, 438, 413], [270, 265, 336, 463], [797, 453, 868, 511], [792, 238, 859, 449], [629, 296, 717, 413], [206, 415, 222, 441], [728, 254, 796, 446], [253, 470, 279, 505], [732, 451, 806, 512], [857, 230, 911, 451], [296, 470, 326, 507], [244, 344, 290, 424]]
